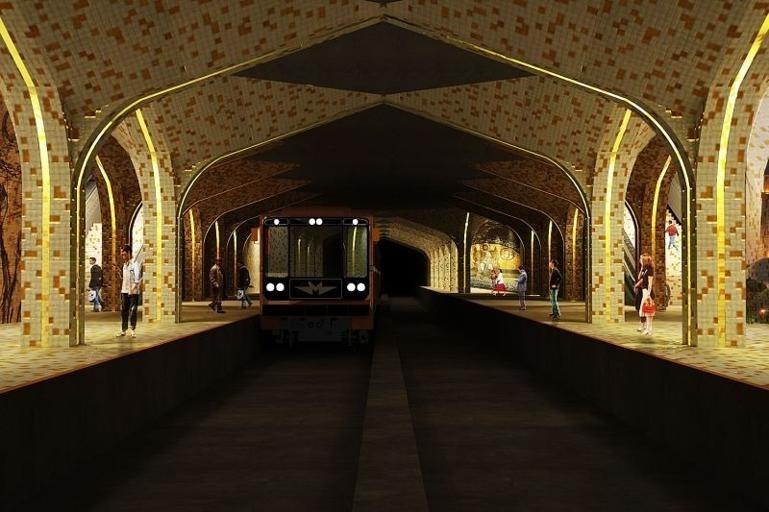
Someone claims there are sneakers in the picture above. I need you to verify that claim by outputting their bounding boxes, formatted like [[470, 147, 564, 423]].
[[116, 327, 136, 338], [207, 300, 254, 313], [633, 325, 654, 337]]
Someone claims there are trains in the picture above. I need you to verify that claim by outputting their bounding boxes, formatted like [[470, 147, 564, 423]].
[[259, 203, 382, 341]]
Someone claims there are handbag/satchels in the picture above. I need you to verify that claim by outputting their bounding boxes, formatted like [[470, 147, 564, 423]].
[[642, 296, 657, 314]]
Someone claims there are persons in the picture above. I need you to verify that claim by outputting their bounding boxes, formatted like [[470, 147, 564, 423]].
[[117, 245, 141, 337], [89, 257, 107, 312], [634, 253, 655, 336], [209, 257, 226, 313], [514, 265, 527, 310], [490, 270, 499, 295], [549, 259, 562, 320], [664, 220, 679, 251], [237, 261, 252, 309], [494, 269, 506, 297]]
